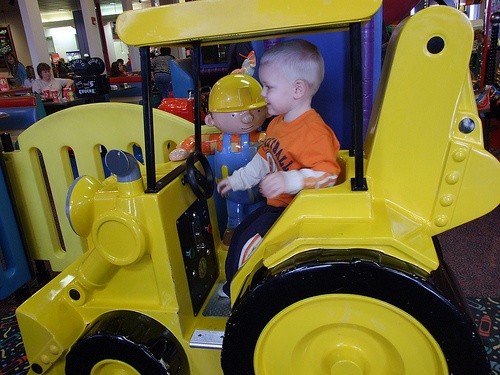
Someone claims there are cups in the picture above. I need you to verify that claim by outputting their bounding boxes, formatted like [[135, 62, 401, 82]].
[[124, 83, 128, 88], [42, 87, 69, 101]]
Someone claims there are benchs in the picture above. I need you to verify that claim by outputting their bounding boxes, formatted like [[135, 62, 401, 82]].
[[107, 75, 142, 104], [0, 94, 48, 137]]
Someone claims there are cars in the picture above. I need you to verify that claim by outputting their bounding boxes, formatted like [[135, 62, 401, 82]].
[[0, 1, 500, 375]]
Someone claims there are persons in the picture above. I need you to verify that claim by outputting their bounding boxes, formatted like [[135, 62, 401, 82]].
[[169, 73, 269, 248], [7, 38, 256, 103], [216, 39, 343, 297]]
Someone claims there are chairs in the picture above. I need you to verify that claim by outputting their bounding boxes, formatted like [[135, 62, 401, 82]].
[[228, 4, 500, 306]]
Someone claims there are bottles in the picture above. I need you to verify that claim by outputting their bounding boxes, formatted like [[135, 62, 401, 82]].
[[76, 81, 96, 94]]
